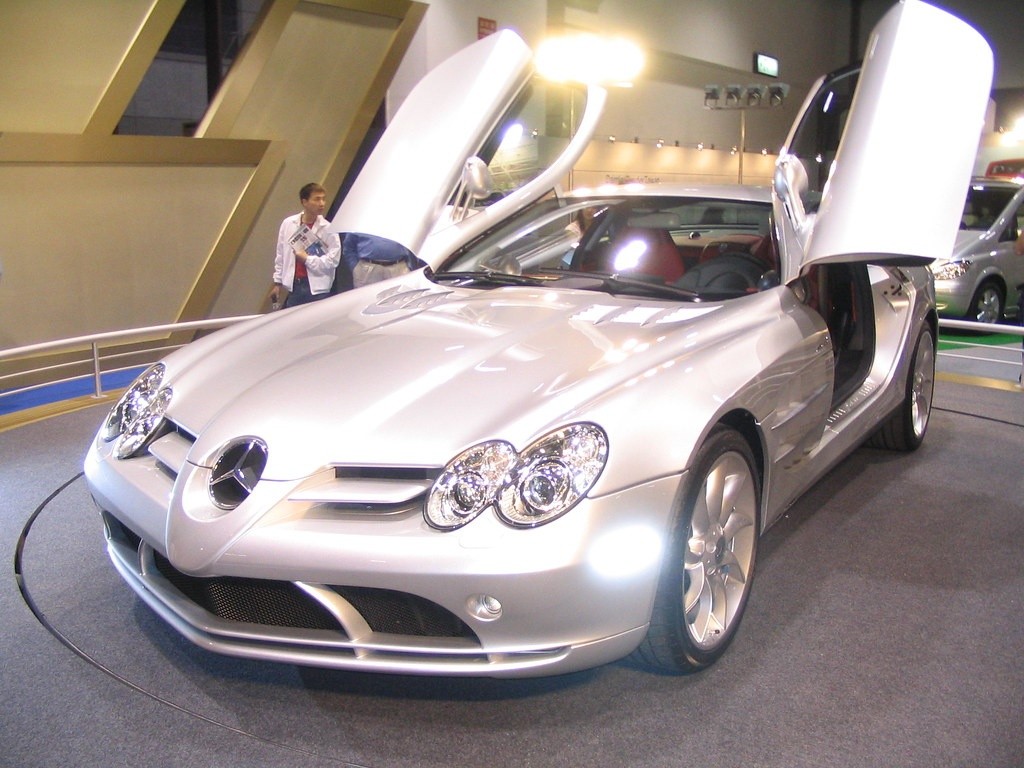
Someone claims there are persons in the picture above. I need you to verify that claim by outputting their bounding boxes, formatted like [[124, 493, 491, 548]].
[[270, 183, 342, 311]]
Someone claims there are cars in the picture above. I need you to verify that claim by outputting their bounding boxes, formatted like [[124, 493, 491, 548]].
[[986, 159, 1024, 180], [918, 178, 1023, 325]]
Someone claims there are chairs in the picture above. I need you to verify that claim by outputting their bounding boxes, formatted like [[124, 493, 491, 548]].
[[700, 236, 835, 326], [605, 226, 682, 285]]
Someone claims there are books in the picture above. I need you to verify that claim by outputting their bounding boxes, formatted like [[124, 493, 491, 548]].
[[288, 225, 331, 259]]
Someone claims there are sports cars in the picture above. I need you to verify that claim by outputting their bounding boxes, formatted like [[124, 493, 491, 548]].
[[81, 0, 995, 690]]
